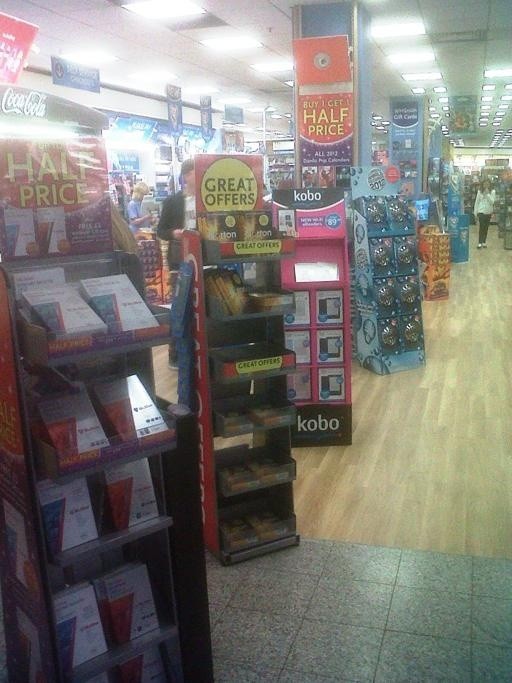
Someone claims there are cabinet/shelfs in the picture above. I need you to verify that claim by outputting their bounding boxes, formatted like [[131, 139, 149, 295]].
[[276, 190, 351, 446], [182, 210, 301, 566], [353, 195, 425, 377], [0, 251, 215, 683], [462, 166, 512, 251]]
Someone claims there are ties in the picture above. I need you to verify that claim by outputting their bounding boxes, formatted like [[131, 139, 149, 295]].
[[477, 242, 482, 248]]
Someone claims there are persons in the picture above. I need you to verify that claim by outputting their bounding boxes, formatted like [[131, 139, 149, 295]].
[[474, 178, 496, 250], [127, 183, 154, 238], [158, 158, 196, 367]]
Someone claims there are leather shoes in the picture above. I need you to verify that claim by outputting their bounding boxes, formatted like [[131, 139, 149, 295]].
[[483, 243, 487, 248]]
